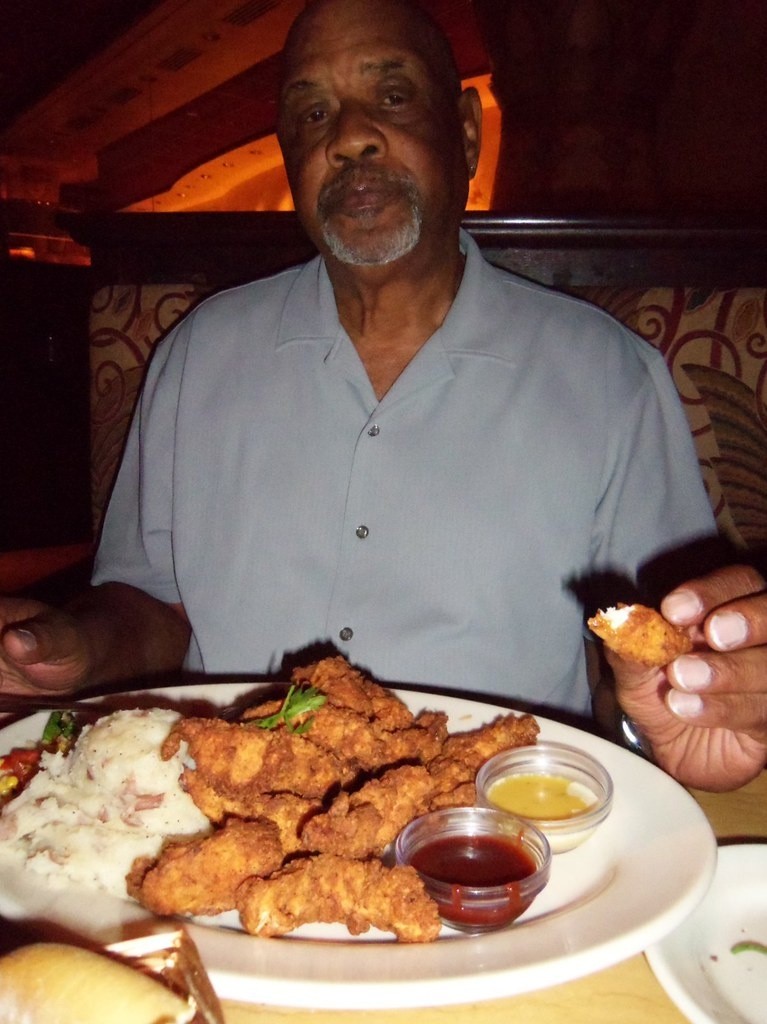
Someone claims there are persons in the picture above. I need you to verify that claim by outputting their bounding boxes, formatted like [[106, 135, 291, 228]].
[[0, 1, 767, 795]]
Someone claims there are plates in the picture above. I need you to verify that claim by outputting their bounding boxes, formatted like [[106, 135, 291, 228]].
[[0, 681, 718, 1008], [642, 842, 767, 1024]]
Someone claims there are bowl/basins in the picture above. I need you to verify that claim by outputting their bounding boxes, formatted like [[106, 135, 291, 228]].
[[394, 806, 552, 935], [474, 745, 615, 856]]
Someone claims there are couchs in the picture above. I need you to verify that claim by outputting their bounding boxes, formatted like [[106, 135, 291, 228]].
[[88, 283, 767, 582]]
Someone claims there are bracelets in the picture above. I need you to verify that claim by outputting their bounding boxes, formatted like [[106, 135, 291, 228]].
[[620, 713, 652, 763]]
